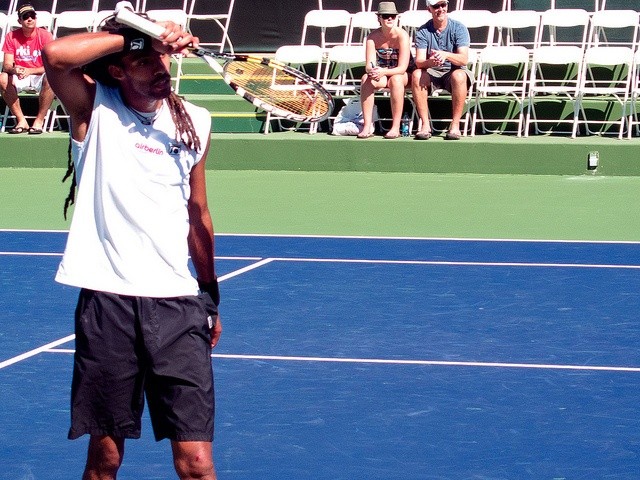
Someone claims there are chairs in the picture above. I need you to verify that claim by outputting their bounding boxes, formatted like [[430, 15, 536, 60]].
[[483, 10, 541, 99], [550, 1, 598, 48], [52, 10, 97, 40], [596, 1, 640, 50], [410, 0, 460, 47], [142, 10, 188, 95], [139, 0, 187, 55], [93, 0, 140, 54], [264, 45, 323, 134], [0, 12, 8, 75], [318, 0, 363, 48], [363, 0, 415, 46], [7, 11, 54, 96], [92, 11, 140, 35], [530, 9, 590, 99], [284, 9, 351, 96], [524, 46, 584, 138], [366, 47, 418, 137], [571, 47, 634, 140], [417, 47, 477, 136], [388, 10, 440, 98], [506, 0, 555, 46], [579, 10, 640, 101], [0, 42, 56, 132], [631, 14, 640, 101], [340, 12, 398, 97], [628, 50, 640, 140], [6, 0, 57, 37], [177, 1, 235, 56], [49, 42, 102, 132], [434, 10, 493, 98], [470, 45, 529, 138], [314, 45, 371, 135], [48, 0, 98, 34], [459, 0, 506, 46]]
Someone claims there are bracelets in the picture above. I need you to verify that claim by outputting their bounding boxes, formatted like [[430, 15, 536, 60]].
[[195, 276, 219, 308], [120, 26, 154, 53]]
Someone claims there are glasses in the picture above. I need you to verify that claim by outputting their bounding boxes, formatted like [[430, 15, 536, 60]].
[[22, 12, 36, 20], [378, 15, 396, 19], [430, 3, 447, 8]]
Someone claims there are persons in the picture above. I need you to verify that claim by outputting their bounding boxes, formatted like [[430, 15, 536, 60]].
[[356, 2, 417, 138], [412, 0, 475, 140], [0, 5, 55, 135], [42, 15, 220, 479]]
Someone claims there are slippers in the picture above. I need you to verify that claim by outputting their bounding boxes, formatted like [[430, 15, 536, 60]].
[[446, 122, 461, 140], [358, 134, 375, 138], [9, 127, 28, 133], [415, 131, 431, 140], [385, 131, 401, 139], [29, 127, 42, 133]]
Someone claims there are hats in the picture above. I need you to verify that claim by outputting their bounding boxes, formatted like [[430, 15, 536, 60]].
[[375, 2, 399, 15], [18, 3, 37, 17], [426, 0, 449, 7]]
